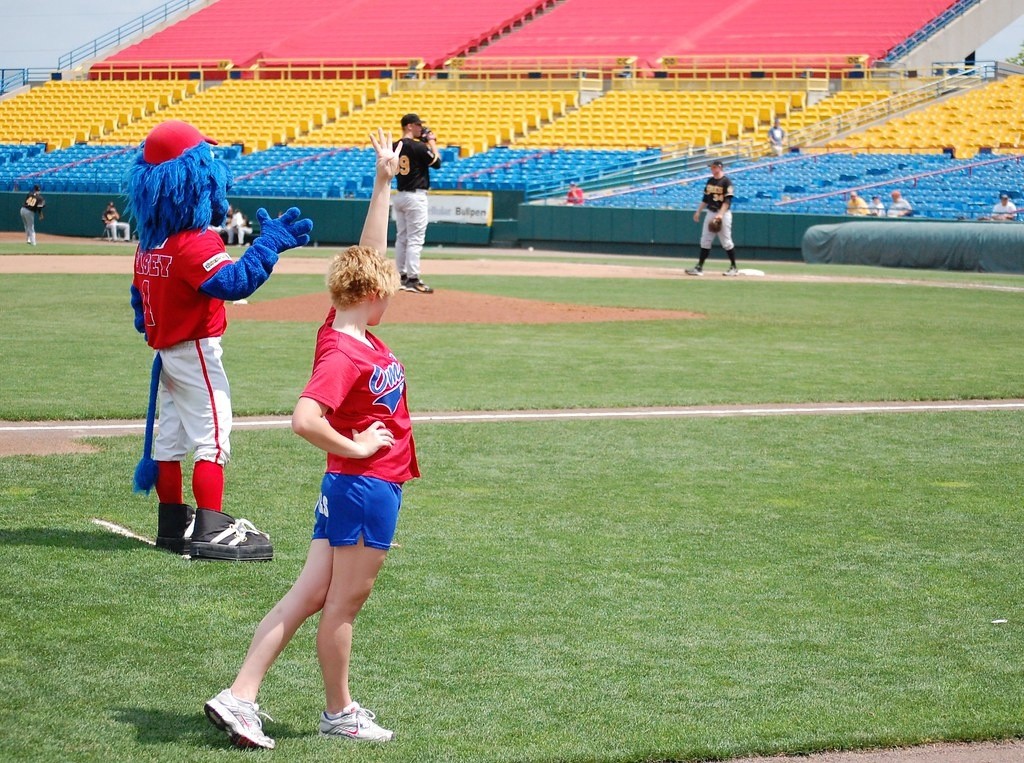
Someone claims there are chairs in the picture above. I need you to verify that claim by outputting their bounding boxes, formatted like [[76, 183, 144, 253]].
[[101, 217, 113, 241], [1, 0, 1022, 222]]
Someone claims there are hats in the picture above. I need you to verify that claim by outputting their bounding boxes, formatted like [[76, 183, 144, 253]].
[[708, 161, 723, 168], [891, 190, 899, 197], [569, 181, 575, 186], [1000, 192, 1007, 199], [401, 114, 426, 127], [109, 202, 114, 207]]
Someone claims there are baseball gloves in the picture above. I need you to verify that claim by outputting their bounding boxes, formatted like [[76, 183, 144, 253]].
[[418, 127, 436, 142], [709, 218, 721, 232]]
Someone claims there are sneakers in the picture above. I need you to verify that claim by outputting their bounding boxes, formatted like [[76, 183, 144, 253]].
[[204, 689, 276, 750], [399, 275, 408, 290], [406, 279, 433, 294], [319, 701, 396, 742]]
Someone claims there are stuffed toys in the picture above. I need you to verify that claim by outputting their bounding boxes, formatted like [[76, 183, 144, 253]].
[[120, 120, 313, 563]]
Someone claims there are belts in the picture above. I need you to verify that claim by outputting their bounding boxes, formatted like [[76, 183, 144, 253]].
[[398, 189, 415, 193]]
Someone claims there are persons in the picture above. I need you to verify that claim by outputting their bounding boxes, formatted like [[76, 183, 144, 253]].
[[768, 117, 787, 156], [992, 194, 1018, 221], [21, 184, 46, 246], [567, 184, 585, 207], [102, 202, 132, 242], [848, 191, 912, 217], [208, 206, 282, 247], [392, 114, 441, 294], [204, 127, 421, 752], [685, 159, 741, 277]]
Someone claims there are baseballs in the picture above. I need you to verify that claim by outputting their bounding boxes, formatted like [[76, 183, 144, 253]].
[[527, 246, 534, 251], [438, 244, 443, 248]]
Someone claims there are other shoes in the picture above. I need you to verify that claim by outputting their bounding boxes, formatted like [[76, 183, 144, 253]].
[[723, 268, 739, 277], [685, 268, 703, 277]]
